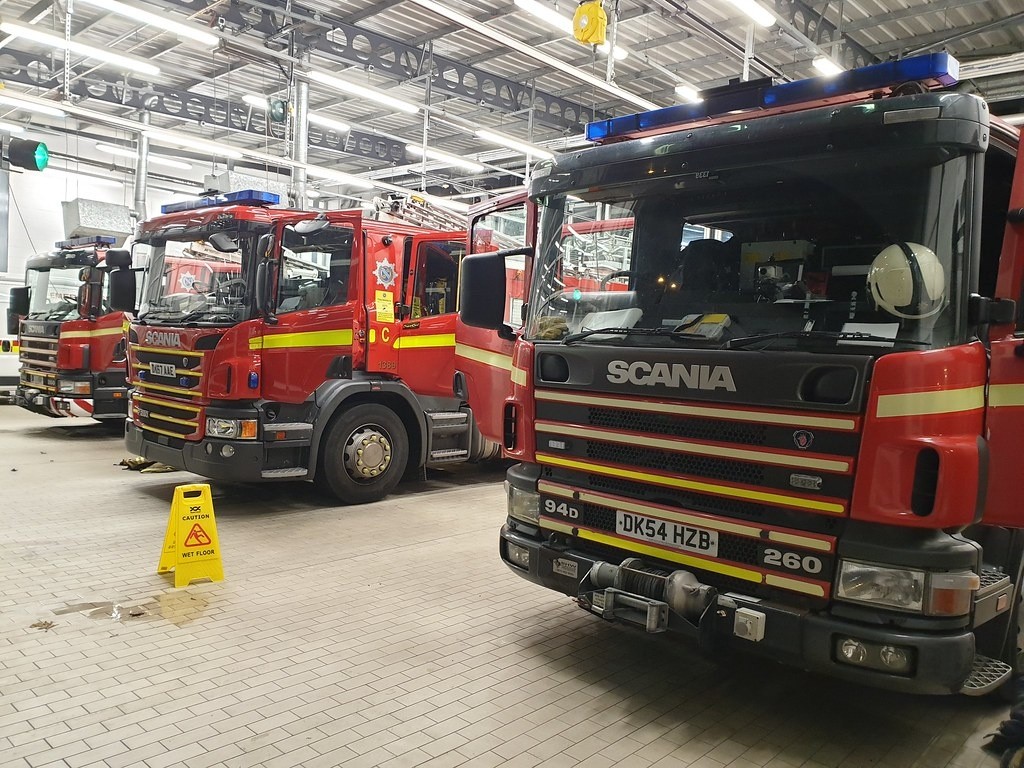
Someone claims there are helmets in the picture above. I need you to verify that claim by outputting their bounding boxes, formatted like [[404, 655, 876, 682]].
[[865, 240, 946, 330]]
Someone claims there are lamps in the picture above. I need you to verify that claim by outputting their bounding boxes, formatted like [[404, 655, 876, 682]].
[[1, 0, 843, 213]]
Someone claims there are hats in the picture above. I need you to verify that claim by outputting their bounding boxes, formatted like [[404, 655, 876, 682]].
[[431, 264, 451, 281]]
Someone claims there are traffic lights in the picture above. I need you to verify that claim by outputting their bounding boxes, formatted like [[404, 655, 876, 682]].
[[8, 133, 52, 174]]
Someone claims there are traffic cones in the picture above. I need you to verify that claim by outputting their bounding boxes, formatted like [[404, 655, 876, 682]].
[[121, 187, 533, 508]]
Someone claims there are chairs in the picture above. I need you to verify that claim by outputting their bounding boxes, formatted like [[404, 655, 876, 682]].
[[652, 237, 745, 324], [810, 236, 890, 323], [302, 250, 350, 311]]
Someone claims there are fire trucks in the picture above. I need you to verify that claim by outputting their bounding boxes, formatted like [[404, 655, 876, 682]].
[[455, 46, 1024, 703], [8, 234, 241, 439]]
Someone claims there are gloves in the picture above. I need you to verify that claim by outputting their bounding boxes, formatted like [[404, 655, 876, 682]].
[[535, 314, 568, 339]]
